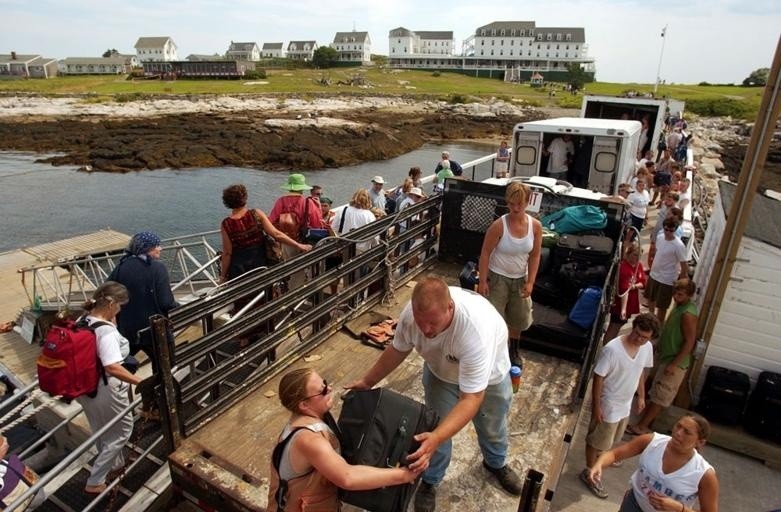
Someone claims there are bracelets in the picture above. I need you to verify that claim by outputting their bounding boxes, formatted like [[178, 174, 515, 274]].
[[682, 503, 684, 512]]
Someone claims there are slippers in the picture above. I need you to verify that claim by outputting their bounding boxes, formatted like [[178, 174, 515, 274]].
[[135, 406, 163, 422], [626, 424, 649, 435], [80, 482, 114, 498]]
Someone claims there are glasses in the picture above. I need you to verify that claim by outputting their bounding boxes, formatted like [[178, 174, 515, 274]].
[[665, 228, 675, 232], [302, 379, 327, 401]]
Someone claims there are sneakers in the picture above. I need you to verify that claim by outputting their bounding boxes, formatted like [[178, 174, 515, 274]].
[[597, 450, 623, 466], [581, 468, 609, 498]]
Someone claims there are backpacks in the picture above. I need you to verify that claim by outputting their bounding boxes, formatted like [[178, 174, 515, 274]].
[[38, 313, 112, 404], [556, 232, 613, 266], [569, 286, 603, 331], [279, 196, 302, 239]]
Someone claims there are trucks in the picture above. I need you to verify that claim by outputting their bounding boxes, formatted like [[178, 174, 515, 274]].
[[578, 94, 668, 163], [140, 175, 643, 512], [508, 117, 643, 198]]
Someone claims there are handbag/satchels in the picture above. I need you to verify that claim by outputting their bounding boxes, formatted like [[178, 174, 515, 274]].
[[300, 196, 330, 243], [538, 206, 608, 235], [251, 208, 285, 266], [459, 261, 480, 291], [653, 169, 671, 186], [0, 453, 41, 512]]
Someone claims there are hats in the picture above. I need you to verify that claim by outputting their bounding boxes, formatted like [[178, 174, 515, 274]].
[[371, 176, 386, 184], [280, 174, 314, 191], [409, 188, 426, 198], [618, 186, 634, 193]]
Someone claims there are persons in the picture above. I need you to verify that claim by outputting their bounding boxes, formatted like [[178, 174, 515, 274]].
[[266, 368, 418, 512], [106, 232, 184, 420], [579, 311, 662, 499], [479, 181, 543, 369], [220, 150, 465, 360], [76, 281, 143, 496], [0, 436, 41, 512], [342, 277, 523, 512], [590, 412, 719, 512], [495, 141, 510, 179], [624, 278, 699, 436], [542, 82, 697, 324]]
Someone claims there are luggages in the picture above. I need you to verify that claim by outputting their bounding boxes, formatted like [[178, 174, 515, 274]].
[[745, 370, 781, 444], [532, 247, 615, 309], [337, 387, 440, 512], [696, 365, 751, 427]]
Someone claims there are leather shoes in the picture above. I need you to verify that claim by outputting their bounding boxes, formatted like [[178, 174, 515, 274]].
[[483, 458, 523, 495], [510, 349, 522, 369], [415, 480, 437, 512]]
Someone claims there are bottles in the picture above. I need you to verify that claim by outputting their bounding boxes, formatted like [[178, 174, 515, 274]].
[[510, 365, 521, 395]]
